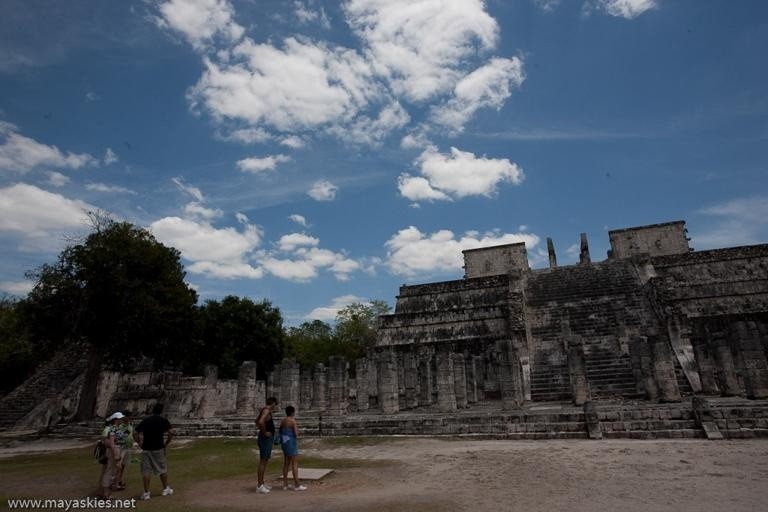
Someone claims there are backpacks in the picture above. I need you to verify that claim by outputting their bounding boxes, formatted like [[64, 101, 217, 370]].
[[93, 439, 108, 465]]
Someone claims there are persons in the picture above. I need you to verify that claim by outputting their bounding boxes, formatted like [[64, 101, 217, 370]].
[[132, 402, 175, 500], [278, 405, 308, 492], [95, 410, 129, 501], [254, 396, 278, 494], [113, 410, 135, 490]]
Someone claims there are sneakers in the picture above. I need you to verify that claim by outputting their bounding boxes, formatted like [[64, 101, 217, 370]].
[[139, 491, 152, 500], [159, 485, 174, 496], [255, 483, 273, 493], [294, 483, 307, 491], [283, 483, 292, 490]]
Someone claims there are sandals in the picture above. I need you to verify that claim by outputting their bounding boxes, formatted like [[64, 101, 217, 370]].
[[113, 480, 128, 490]]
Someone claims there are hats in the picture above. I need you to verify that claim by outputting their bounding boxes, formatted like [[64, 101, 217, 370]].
[[105, 411, 126, 421]]
